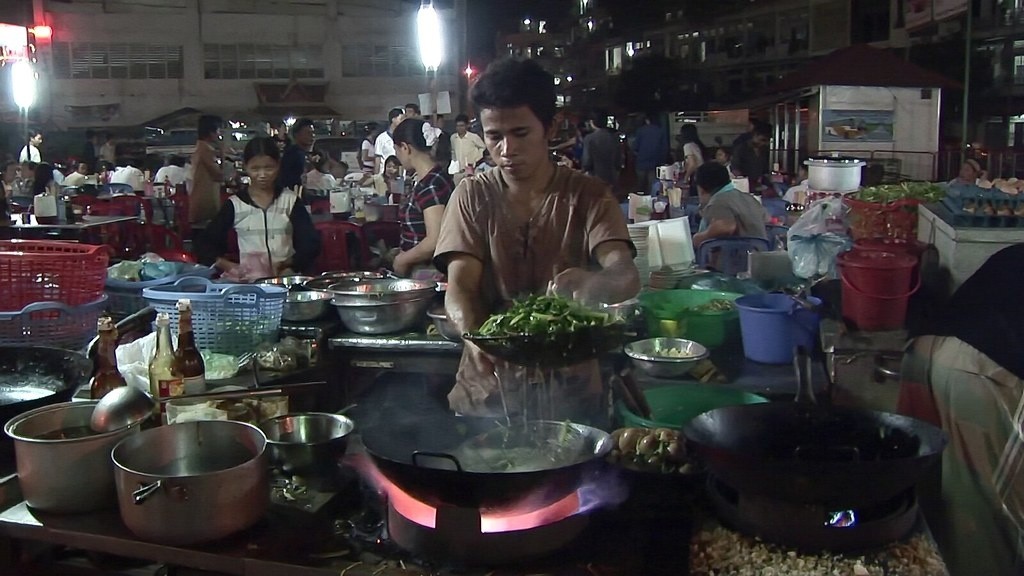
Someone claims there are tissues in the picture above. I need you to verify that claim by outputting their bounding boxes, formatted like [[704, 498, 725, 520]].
[[33, 187, 58, 224], [380, 193, 399, 221]]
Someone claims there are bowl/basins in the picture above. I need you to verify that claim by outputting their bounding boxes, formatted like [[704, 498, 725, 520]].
[[259, 412, 355, 475], [625, 337, 711, 379], [254, 270, 463, 343]]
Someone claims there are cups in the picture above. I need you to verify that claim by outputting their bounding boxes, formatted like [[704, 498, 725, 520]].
[[19, 211, 30, 224], [73, 209, 83, 224]]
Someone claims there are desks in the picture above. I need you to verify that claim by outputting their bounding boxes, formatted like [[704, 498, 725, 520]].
[[4, 212, 138, 254], [189, 213, 365, 229]]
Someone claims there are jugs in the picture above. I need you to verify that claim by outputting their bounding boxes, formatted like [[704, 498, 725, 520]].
[[628, 191, 652, 222], [656, 164, 676, 181]]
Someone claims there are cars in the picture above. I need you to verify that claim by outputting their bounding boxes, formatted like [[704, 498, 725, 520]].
[[145, 126, 199, 160], [229, 129, 263, 151]]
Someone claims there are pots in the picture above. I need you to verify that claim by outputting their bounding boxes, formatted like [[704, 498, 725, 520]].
[[803, 156, 867, 190], [361, 348, 951, 517], [1, 305, 267, 546]]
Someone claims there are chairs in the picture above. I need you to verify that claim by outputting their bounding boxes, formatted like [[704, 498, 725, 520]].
[[306, 199, 403, 274], [698, 224, 790, 275], [68, 194, 197, 267]]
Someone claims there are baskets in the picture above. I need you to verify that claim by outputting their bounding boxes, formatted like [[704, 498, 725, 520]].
[[0, 238, 115, 349], [140, 276, 288, 354]]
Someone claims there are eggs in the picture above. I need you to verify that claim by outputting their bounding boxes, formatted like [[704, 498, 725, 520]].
[[604, 426, 688, 465]]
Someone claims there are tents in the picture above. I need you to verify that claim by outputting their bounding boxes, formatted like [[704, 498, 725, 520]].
[[0, 22, 32, 162]]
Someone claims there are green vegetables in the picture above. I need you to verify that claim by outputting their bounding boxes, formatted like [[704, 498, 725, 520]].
[[691, 299, 736, 311], [849, 182, 946, 225], [473, 294, 634, 360], [481, 418, 571, 470]]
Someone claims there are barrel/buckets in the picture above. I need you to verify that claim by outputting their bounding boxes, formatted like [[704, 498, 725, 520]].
[[638, 191, 927, 364], [619, 385, 771, 431]]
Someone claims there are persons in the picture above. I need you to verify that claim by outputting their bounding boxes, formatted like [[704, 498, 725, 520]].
[[193, 136, 321, 281], [431, 57, 642, 424], [899, 241, 1024, 576], [958, 159, 980, 182], [548, 110, 774, 270], [393, 117, 456, 279], [0, 103, 486, 264]]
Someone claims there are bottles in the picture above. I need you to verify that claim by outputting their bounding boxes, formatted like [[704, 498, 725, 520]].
[[175, 301, 207, 394], [57, 194, 75, 225], [148, 313, 184, 425], [354, 176, 404, 222], [90, 313, 127, 400]]
